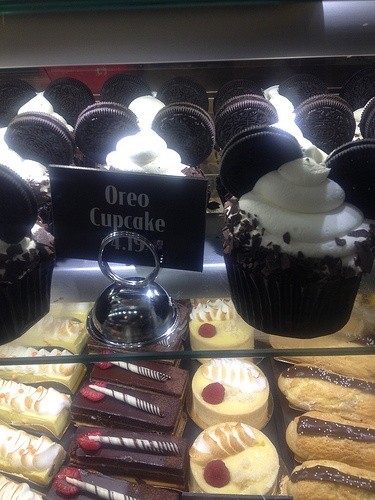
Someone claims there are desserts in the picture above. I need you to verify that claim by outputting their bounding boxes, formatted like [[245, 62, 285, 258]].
[[0, 298, 282, 499], [0, 74, 375, 345]]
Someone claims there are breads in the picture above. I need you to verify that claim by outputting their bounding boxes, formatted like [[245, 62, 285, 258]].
[[267, 291, 375, 500]]
